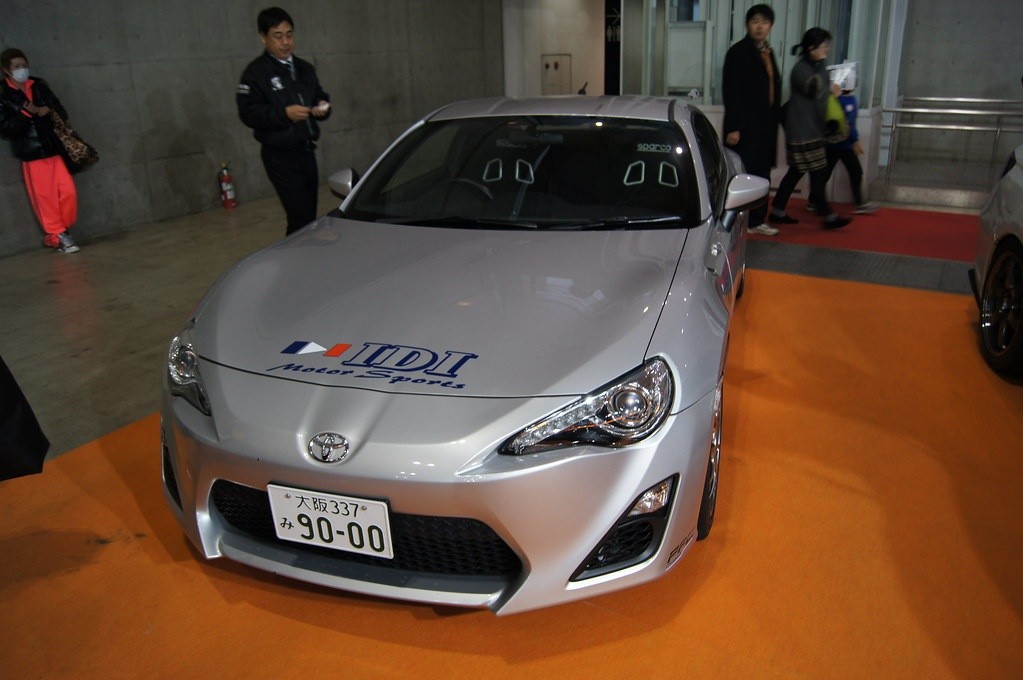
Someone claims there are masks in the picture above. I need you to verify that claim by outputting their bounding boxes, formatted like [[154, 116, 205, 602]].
[[9, 69, 30, 84]]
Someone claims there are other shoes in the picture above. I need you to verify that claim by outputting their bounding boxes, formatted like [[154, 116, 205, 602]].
[[853, 202, 880, 214], [826, 216, 852, 229], [768, 212, 799, 224], [807, 203, 820, 211]]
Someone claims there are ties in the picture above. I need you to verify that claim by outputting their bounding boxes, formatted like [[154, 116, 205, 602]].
[[285, 60, 315, 137]]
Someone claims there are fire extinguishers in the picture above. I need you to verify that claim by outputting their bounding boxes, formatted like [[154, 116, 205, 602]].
[[217, 159, 237, 209]]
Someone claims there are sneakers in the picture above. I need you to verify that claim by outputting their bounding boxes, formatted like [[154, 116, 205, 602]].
[[56, 231, 80, 253], [747, 222, 779, 236]]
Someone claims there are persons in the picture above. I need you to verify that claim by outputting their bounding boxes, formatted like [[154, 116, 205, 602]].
[[236, 5, 333, 240], [768, 26, 880, 231], [0, 47, 80, 254], [722, 4, 783, 236], [50, 249, 99, 350]]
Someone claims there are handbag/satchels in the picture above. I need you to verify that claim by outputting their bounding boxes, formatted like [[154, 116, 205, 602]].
[[51, 111, 98, 174], [825, 94, 846, 134]]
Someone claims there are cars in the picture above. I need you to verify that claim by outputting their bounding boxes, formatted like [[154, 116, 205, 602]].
[[163, 90, 772, 617], [968, 145, 1023, 387]]
